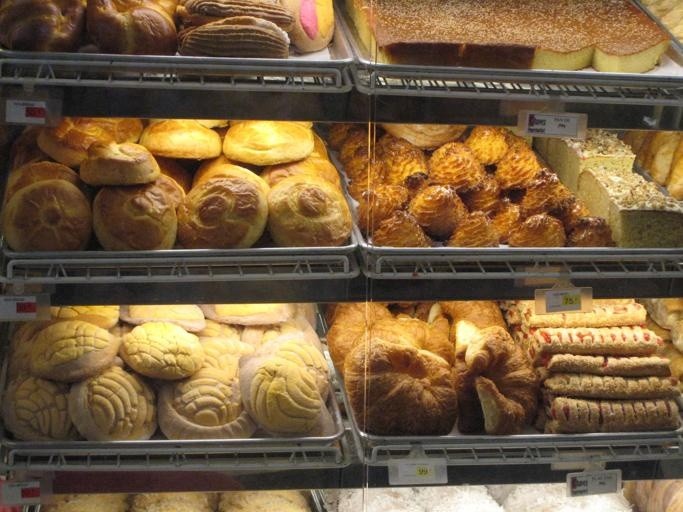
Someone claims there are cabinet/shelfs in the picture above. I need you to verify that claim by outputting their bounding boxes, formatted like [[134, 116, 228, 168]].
[[0, 0, 680, 512]]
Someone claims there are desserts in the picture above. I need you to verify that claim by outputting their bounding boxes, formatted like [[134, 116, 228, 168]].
[[1, 95, 682, 273], [0, 294, 683, 512], [0, 0, 683, 78]]
